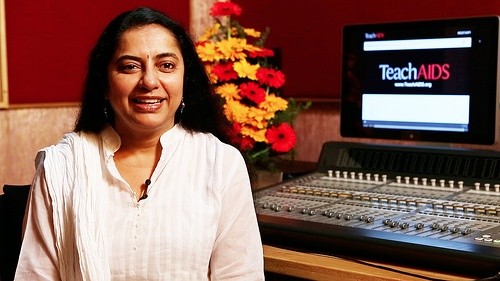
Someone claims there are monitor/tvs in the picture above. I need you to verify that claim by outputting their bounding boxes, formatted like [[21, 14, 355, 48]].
[[339, 16, 500, 146]]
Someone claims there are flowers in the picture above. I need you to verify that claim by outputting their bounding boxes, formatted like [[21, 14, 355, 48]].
[[193, 2, 313, 170]]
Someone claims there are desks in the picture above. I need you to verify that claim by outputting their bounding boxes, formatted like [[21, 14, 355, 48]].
[[257, 225, 500, 281]]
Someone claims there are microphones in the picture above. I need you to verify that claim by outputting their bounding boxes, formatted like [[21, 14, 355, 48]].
[[138, 179, 151, 200]]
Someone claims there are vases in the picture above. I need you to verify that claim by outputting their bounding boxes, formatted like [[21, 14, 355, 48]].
[[246, 171, 283, 194]]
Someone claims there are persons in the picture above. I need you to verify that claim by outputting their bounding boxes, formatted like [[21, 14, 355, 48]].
[[13, 7, 265, 281]]
